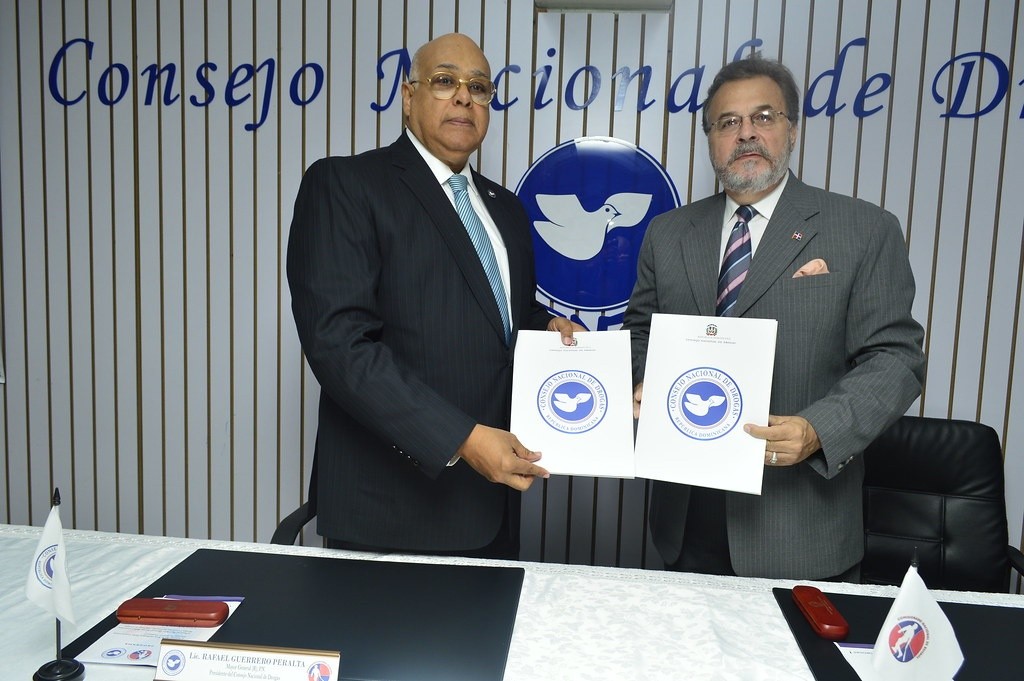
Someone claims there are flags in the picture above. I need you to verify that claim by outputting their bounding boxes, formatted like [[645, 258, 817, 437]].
[[871, 567, 964, 681], [25, 506, 74, 625]]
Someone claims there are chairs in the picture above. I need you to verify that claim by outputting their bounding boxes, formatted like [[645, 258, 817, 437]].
[[860, 417, 1024, 593]]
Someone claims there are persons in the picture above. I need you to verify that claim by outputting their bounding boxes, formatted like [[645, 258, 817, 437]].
[[287, 33, 588, 561], [619, 58, 927, 584]]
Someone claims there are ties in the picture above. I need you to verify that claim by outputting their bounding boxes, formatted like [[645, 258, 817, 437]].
[[716, 204, 759, 317], [447, 174, 511, 348]]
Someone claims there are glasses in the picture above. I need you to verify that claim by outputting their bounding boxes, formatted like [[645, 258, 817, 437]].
[[708, 109, 792, 131], [409, 72, 497, 105]]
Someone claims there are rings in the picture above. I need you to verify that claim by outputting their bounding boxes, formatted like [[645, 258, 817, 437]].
[[770, 452, 777, 464]]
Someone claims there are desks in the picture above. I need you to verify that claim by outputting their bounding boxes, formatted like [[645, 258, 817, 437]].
[[0, 523, 1024, 681]]
